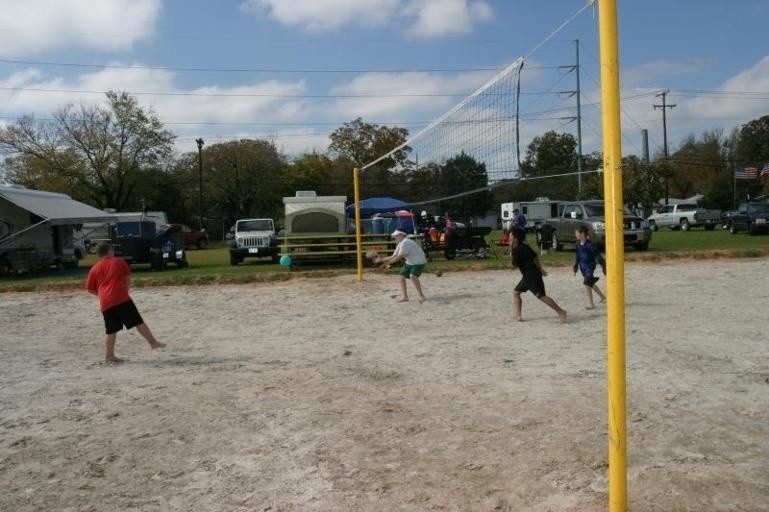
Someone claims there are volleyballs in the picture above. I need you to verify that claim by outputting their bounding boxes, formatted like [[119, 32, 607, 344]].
[[366, 250, 377, 260], [436, 270, 442, 277], [280, 256, 291, 266]]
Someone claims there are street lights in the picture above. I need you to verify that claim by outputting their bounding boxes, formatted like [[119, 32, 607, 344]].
[[193, 136, 206, 231]]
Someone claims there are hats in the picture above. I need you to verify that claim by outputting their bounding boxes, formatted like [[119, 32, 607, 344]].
[[391, 230, 407, 236]]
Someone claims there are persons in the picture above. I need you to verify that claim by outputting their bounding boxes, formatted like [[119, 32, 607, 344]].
[[506, 225, 566, 323], [512, 208, 527, 232], [372, 227, 427, 303], [573, 226, 606, 310], [85, 242, 167, 363]]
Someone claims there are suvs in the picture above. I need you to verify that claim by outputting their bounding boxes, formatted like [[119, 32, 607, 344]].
[[223, 216, 280, 266]]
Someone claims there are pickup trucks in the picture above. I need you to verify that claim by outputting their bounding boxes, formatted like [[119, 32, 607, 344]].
[[641, 201, 724, 231], [537, 196, 655, 256], [719, 199, 769, 235]]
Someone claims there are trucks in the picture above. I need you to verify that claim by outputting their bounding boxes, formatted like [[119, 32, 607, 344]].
[[71, 206, 167, 255]]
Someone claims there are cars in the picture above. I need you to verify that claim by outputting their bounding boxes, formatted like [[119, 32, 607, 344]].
[[107, 223, 189, 271], [154, 221, 208, 251]]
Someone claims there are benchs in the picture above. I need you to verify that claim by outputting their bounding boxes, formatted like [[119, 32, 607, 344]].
[[277, 233, 397, 269], [0, 246, 53, 278]]
